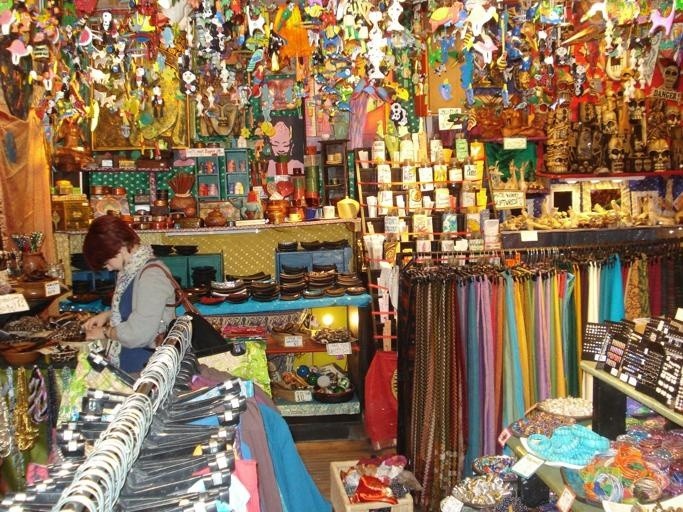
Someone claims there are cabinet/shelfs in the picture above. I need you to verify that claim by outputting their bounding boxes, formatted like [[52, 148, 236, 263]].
[[44, 136, 375, 446], [502, 354, 683, 511]]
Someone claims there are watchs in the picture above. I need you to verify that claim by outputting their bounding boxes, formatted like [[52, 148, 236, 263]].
[[102, 325, 112, 340]]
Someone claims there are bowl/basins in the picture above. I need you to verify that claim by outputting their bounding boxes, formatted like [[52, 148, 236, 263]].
[[3, 342, 42, 365], [313, 383, 355, 403], [208, 142, 219, 148], [194, 143, 205, 148]]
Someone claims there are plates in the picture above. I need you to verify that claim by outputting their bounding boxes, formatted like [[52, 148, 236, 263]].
[[471, 453, 518, 481], [172, 264, 366, 305], [68, 252, 90, 270], [67, 293, 99, 303], [276, 239, 348, 252], [150, 244, 199, 255]]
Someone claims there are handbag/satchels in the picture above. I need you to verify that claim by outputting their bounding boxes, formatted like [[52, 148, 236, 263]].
[[137, 262, 228, 353]]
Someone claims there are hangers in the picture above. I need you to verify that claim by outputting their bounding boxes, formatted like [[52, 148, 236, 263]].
[[401, 234, 683, 290], [0, 313, 252, 512]]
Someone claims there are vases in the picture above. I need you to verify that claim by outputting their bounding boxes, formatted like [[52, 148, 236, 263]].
[[18, 250, 47, 281]]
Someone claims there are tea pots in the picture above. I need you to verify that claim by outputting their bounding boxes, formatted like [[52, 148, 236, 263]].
[[203, 205, 227, 227], [337, 195, 360, 218]]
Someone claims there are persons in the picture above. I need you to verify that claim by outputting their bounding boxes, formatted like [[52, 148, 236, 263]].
[[81, 214, 178, 371]]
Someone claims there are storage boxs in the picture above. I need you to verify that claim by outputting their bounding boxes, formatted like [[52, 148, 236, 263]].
[[326, 456, 416, 512]]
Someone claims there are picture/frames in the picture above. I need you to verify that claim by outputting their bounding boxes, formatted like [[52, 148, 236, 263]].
[[543, 182, 582, 218], [87, 27, 191, 153], [579, 179, 631, 219], [630, 189, 661, 219]]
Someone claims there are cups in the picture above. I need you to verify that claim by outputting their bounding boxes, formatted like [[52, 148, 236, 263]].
[[320, 133, 328, 140], [198, 159, 247, 195], [330, 178, 337, 186], [323, 206, 334, 218], [224, 142, 231, 150], [327, 152, 341, 162], [270, 147, 322, 224]]
